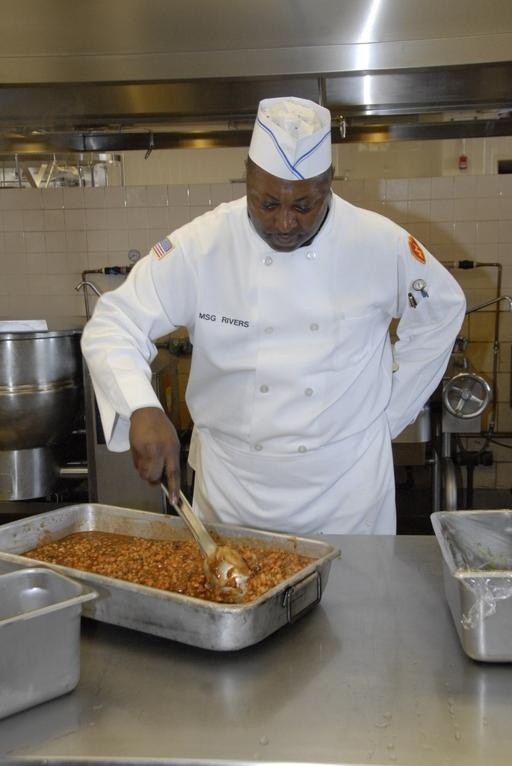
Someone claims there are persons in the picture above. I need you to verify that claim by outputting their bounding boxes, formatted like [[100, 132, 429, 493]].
[[79, 95, 470, 535]]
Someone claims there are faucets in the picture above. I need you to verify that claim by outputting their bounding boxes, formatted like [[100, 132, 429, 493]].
[[73, 282, 103, 297]]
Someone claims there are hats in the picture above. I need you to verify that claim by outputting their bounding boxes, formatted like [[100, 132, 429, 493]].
[[246, 91, 336, 184]]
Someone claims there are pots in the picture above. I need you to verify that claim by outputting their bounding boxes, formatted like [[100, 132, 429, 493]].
[[0, 329, 86, 505]]
